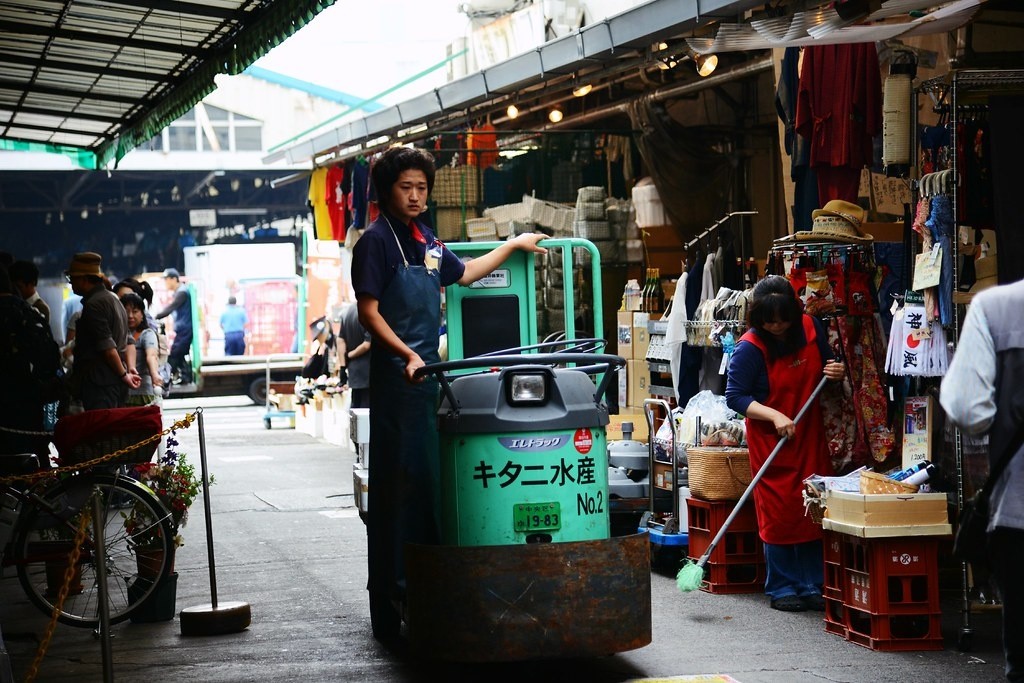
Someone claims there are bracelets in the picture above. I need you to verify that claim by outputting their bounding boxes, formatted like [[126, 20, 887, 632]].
[[121, 372, 126, 377]]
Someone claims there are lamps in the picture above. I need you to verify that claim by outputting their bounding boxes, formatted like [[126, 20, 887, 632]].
[[882, 54, 918, 179], [687, 50, 719, 77]]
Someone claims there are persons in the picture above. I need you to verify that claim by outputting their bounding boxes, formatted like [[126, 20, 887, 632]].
[[336, 303, 370, 460], [725, 274, 844, 612], [939, 280, 1024, 683], [351, 147, 549, 601], [220, 297, 248, 355], [0, 252, 164, 471], [156, 268, 193, 385]]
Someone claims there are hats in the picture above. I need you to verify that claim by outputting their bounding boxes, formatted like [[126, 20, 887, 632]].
[[795, 215, 874, 245], [811, 200, 866, 238], [63, 252, 106, 281], [160, 268, 179, 278]]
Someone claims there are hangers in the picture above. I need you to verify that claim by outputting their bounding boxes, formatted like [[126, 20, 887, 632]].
[[688, 215, 733, 273], [919, 102, 992, 137]]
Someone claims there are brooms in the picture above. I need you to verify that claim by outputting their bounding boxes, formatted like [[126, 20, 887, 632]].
[[675, 355, 841, 592]]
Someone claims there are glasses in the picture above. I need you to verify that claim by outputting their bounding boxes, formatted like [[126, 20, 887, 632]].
[[65, 275, 75, 283]]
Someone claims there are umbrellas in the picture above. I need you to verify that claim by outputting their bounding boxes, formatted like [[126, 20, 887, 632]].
[[912, 170, 961, 330]]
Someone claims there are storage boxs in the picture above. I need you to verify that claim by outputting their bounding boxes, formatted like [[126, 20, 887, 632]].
[[605, 310, 952, 652], [269, 392, 370, 510]]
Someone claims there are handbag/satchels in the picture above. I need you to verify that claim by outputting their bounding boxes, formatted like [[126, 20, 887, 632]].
[[950, 487, 989, 569]]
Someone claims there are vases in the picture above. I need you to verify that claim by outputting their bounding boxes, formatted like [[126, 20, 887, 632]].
[[130, 544, 184, 580], [121, 571, 179, 623]]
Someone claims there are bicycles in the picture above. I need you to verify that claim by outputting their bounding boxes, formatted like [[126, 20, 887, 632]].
[[0, 461, 175, 639]]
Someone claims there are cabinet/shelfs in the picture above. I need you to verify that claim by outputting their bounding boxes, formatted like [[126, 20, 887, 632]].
[[917, 68, 1024, 655]]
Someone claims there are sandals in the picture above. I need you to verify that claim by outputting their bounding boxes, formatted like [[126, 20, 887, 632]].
[[770, 594, 809, 612], [800, 593, 826, 611]]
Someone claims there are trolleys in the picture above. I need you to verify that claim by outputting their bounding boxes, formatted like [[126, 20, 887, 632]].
[[637, 399, 691, 575]]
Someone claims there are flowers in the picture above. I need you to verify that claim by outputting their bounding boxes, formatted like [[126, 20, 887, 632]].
[[119, 429, 216, 557]]
